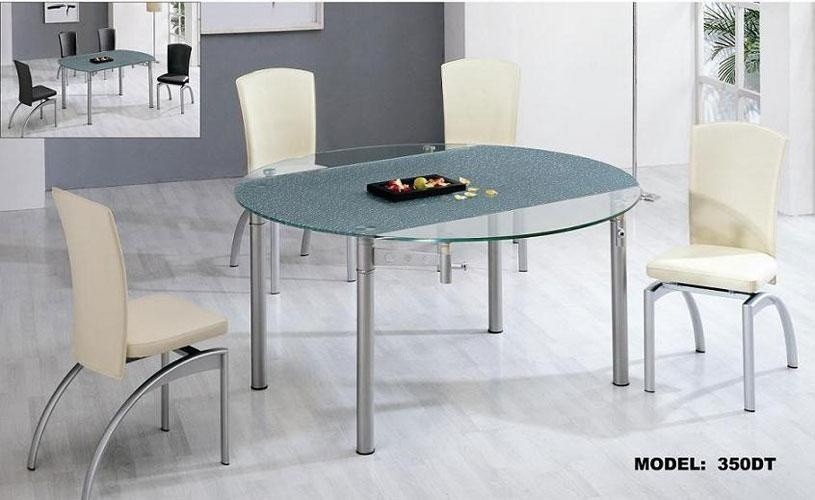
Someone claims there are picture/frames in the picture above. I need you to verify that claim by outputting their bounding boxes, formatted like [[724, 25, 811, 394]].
[[58, 50, 156, 125], [235, 142, 642, 455]]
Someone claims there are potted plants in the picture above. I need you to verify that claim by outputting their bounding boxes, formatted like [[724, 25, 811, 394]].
[[43, 2, 80, 24], [201, 2, 324, 35]]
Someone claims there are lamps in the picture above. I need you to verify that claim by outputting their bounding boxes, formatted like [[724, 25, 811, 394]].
[[641, 121, 798, 411], [9, 60, 57, 138], [28, 186, 232, 500], [227, 65, 359, 294], [439, 56, 529, 282], [157, 43, 194, 114], [56, 31, 87, 83], [97, 27, 115, 80]]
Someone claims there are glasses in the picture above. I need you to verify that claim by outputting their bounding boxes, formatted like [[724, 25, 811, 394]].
[[146, 3, 160, 63]]
